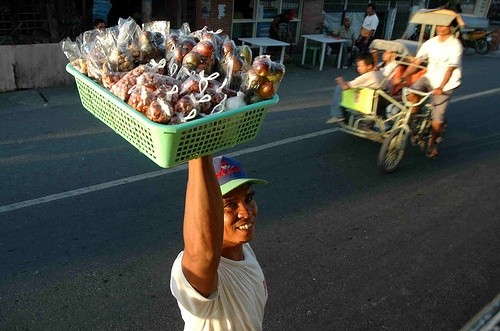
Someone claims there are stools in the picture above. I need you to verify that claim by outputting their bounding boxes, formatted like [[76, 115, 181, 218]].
[[307, 46, 320, 65]]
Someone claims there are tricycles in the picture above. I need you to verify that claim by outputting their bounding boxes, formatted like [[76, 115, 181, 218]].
[[336, 55, 448, 173]]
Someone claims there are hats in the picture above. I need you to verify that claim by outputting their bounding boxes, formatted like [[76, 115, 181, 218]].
[[212, 156, 269, 196]]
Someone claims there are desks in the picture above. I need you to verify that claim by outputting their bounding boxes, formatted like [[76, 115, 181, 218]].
[[238, 37, 291, 65], [302, 34, 348, 71]]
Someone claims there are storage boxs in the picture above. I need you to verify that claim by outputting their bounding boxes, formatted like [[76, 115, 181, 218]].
[[65, 64, 280, 169]]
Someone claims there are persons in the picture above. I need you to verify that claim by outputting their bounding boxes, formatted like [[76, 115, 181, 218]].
[[269, 3, 462, 160], [94, 18, 106, 29], [170, 155, 269, 331]]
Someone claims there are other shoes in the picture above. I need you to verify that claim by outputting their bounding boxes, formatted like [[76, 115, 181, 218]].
[[325, 116, 346, 124], [342, 64, 349, 70]]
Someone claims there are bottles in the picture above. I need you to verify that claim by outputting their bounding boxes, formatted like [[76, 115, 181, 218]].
[[282, 2, 298, 9]]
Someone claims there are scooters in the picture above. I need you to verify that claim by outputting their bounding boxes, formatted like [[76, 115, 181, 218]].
[[460, 29, 495, 55]]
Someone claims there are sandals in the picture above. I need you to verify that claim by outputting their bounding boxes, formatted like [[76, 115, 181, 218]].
[[425, 138, 440, 160]]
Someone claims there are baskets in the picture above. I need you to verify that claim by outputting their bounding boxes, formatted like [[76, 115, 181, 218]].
[[65, 50, 280, 169]]
